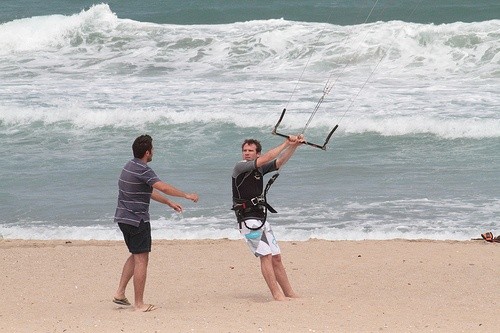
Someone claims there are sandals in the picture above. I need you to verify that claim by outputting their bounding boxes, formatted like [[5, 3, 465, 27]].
[[135, 304, 161, 312], [113, 297, 132, 307]]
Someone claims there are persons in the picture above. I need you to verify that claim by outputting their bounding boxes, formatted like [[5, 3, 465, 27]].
[[111, 135, 199, 312], [231, 134, 310, 300]]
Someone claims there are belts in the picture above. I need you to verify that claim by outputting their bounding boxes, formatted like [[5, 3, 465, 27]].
[[237, 198, 261, 206]]
